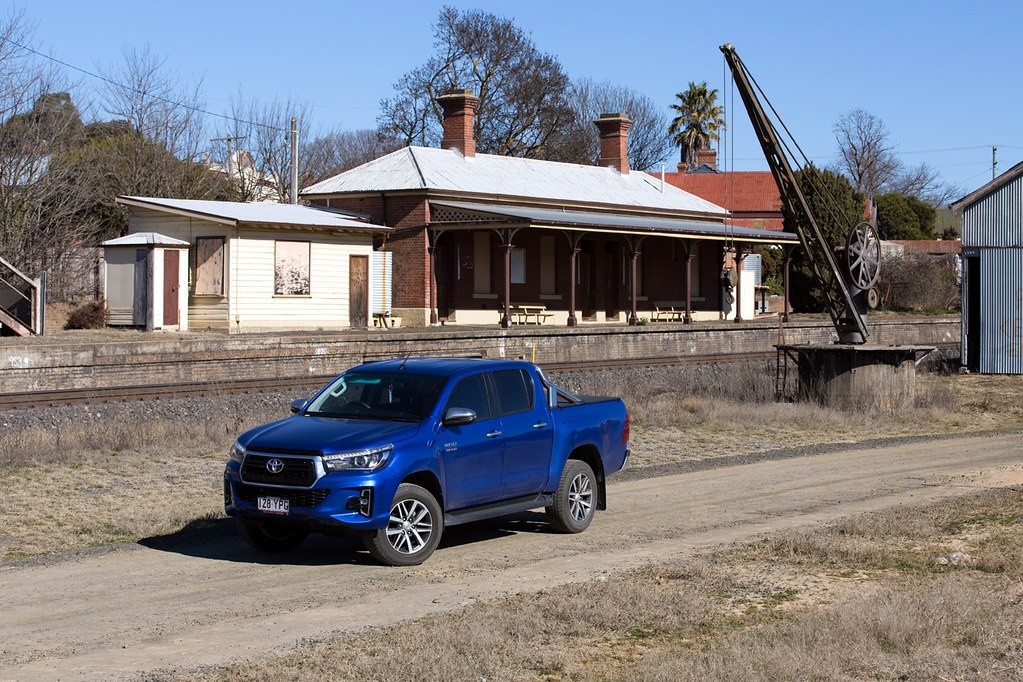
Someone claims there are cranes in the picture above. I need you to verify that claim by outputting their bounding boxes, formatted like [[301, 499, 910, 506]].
[[718, 41, 883, 345]]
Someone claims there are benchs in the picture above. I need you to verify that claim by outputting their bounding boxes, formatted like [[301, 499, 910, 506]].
[[503, 301, 553, 326], [653, 300, 696, 323]]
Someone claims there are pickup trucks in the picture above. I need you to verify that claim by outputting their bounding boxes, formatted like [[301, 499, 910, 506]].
[[222, 354, 632, 568]]
